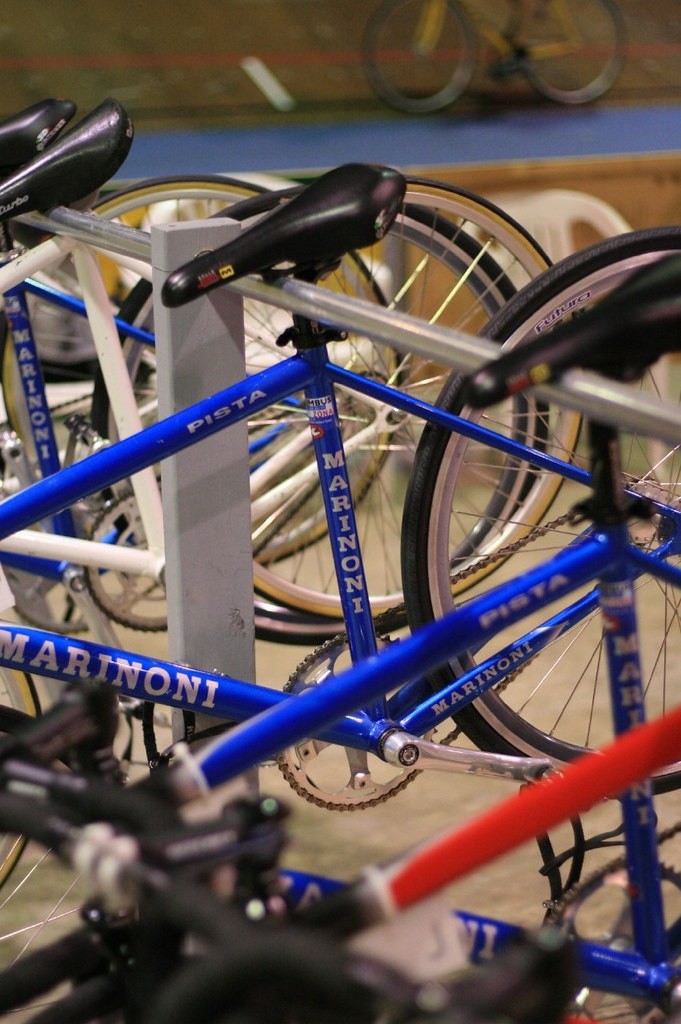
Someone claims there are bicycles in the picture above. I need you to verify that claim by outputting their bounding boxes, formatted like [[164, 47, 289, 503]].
[[361, 1, 629, 115], [1, 93, 681, 1024]]
